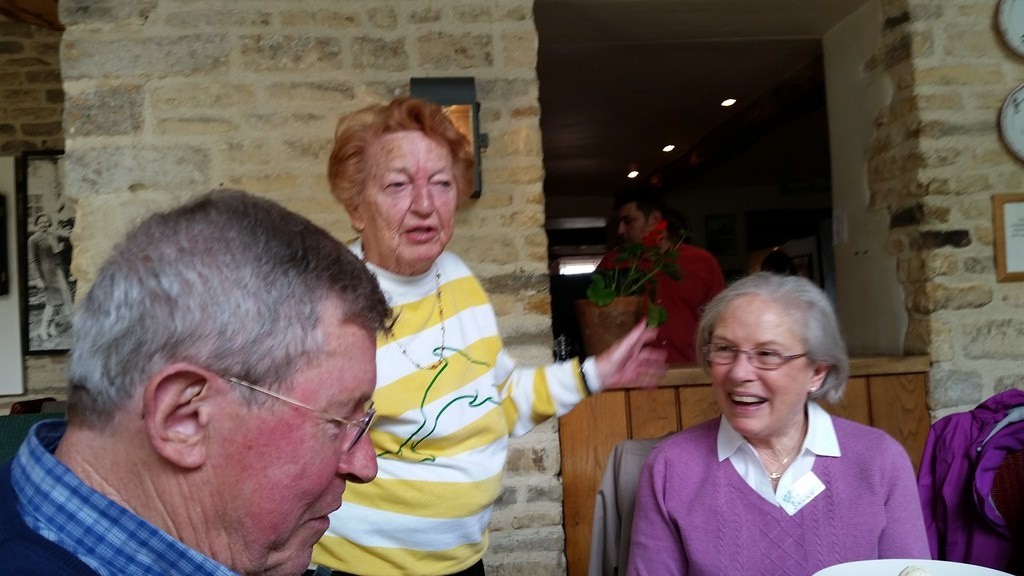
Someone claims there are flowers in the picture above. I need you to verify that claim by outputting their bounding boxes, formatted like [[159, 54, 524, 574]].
[[572, 218, 693, 330]]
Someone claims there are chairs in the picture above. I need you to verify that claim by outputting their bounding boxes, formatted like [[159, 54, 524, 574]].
[[591, 441, 663, 575], [932, 398, 1023, 575]]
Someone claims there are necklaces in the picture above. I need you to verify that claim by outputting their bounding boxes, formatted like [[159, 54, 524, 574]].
[[361, 246, 444, 370], [768, 421, 805, 480], [665, 241, 675, 253]]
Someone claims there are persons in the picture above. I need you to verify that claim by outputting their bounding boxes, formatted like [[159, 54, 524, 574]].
[[625, 271, 931, 576], [0, 189, 392, 576], [308, 100, 667, 576], [590, 191, 724, 364], [28, 213, 74, 341], [548, 254, 586, 354]]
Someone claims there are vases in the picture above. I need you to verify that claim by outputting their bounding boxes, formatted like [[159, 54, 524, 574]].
[[573, 293, 646, 358]]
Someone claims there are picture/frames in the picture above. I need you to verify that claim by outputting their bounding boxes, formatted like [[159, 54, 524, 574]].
[[992, 193, 1024, 284], [20, 151, 77, 354]]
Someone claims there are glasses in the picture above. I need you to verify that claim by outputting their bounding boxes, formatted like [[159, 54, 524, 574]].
[[226, 373, 379, 454], [701, 342, 808, 370]]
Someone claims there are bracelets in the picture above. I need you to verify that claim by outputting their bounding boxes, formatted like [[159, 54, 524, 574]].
[[578, 354, 593, 396]]
